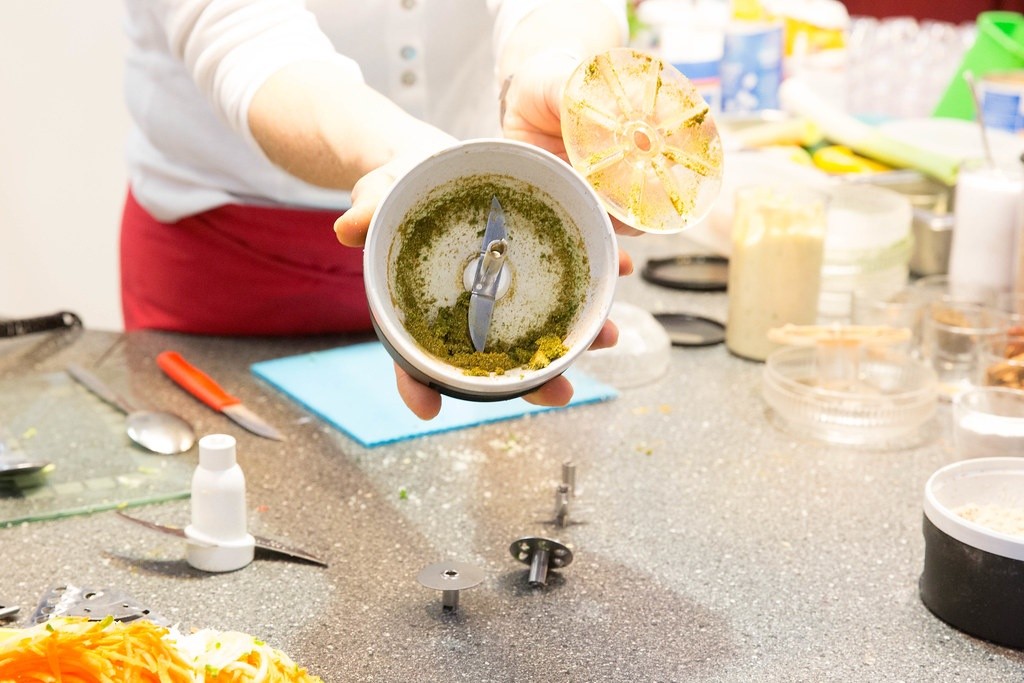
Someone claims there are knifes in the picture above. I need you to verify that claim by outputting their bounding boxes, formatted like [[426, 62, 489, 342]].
[[156, 350, 288, 442]]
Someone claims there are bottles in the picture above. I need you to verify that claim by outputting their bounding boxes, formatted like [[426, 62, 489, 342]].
[[183, 434, 256, 573]]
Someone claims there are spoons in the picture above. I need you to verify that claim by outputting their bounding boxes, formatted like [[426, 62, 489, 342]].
[[63, 361, 197, 456], [0, 442, 50, 474]]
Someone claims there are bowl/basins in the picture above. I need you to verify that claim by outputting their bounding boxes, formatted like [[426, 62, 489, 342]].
[[919, 455, 1024, 655], [762, 185, 937, 446], [363, 138, 619, 404]]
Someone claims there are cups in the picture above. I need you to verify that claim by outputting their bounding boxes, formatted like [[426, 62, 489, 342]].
[[723, 187, 828, 361], [949, 183, 1024, 457]]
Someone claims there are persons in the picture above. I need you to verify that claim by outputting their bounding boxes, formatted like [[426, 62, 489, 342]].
[[119, 0, 716, 421]]
